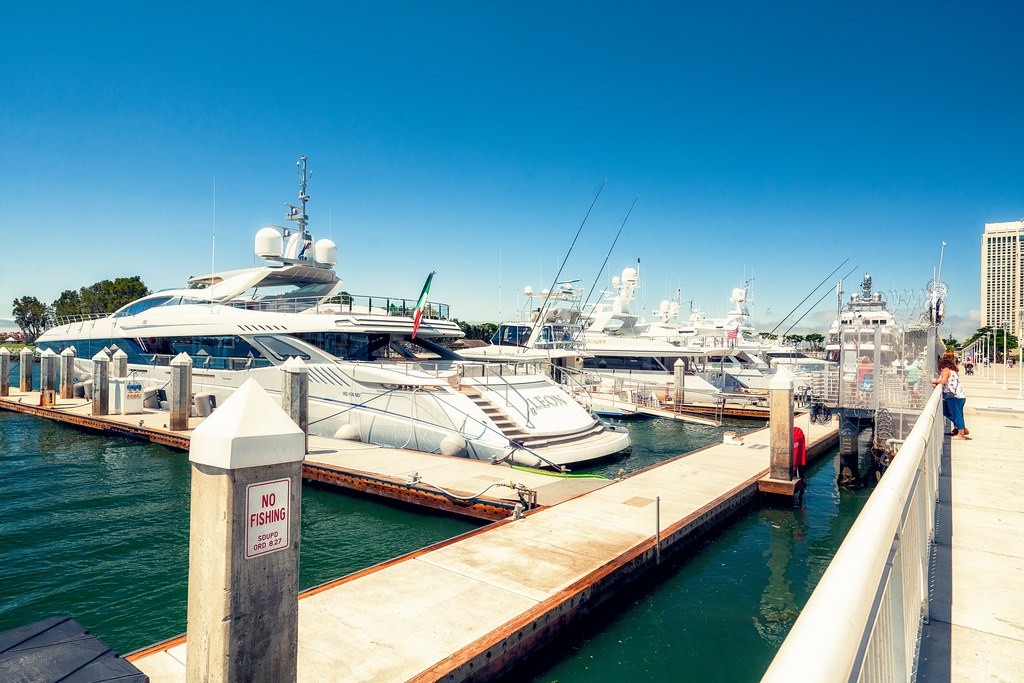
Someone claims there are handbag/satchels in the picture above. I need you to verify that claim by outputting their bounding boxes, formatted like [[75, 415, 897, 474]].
[[944, 392, 956, 399]]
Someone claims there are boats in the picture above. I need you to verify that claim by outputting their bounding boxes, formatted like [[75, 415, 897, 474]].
[[33, 155, 633, 472], [410, 176, 901, 419]]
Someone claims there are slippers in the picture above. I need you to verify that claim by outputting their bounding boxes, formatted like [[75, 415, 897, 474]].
[[950, 435, 966, 441]]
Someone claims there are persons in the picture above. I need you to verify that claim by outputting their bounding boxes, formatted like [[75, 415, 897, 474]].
[[931, 351, 959, 436], [955, 356, 1015, 376], [854, 348, 927, 408], [930, 356, 969, 441]]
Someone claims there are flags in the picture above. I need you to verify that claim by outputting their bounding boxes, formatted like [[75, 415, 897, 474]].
[[411, 272, 434, 340]]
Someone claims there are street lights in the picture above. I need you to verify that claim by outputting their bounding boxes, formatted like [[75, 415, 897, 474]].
[[978, 337, 983, 374], [980, 335, 987, 376], [1000, 319, 1012, 387], [990, 327, 1000, 382], [1014, 306, 1024, 396], [984, 331, 994, 379], [961, 340, 979, 373]]
